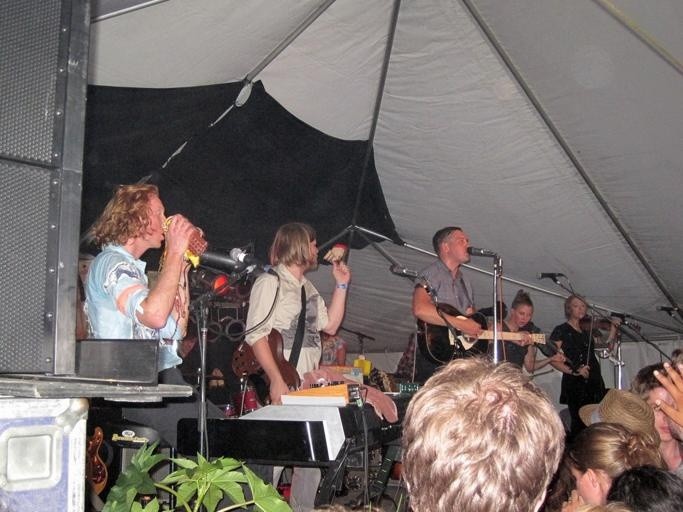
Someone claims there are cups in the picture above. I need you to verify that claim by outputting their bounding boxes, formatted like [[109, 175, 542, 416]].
[[162, 218, 206, 257]]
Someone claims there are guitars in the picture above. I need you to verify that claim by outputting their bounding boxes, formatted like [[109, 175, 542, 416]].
[[86, 427, 108, 499], [232, 327, 299, 401], [418, 299, 547, 363]]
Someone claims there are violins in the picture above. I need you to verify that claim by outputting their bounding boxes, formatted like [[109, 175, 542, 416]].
[[579, 312, 643, 332]]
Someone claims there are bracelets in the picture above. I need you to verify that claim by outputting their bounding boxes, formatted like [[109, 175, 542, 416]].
[[333, 282, 349, 290]]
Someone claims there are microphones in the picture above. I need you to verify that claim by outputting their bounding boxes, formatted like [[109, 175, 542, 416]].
[[230, 247, 276, 279], [535, 271, 562, 281], [656, 305, 680, 313], [466, 246, 495, 258], [388, 264, 421, 281]]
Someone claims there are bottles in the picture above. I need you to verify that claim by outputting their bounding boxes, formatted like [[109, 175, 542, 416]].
[[240, 374, 257, 415], [223, 405, 238, 419]]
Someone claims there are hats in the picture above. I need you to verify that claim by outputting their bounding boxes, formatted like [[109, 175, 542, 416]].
[[576, 387, 662, 450]]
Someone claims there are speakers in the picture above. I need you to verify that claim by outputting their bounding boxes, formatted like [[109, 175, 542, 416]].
[[0, 1, 90, 375]]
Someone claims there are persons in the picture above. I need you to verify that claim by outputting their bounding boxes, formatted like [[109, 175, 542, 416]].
[[490, 289, 564, 377], [548, 294, 620, 435], [178, 332, 226, 378], [237, 220, 350, 512], [403, 355, 567, 511], [411, 227, 536, 379], [78, 183, 253, 511], [559, 350, 683, 512]]
